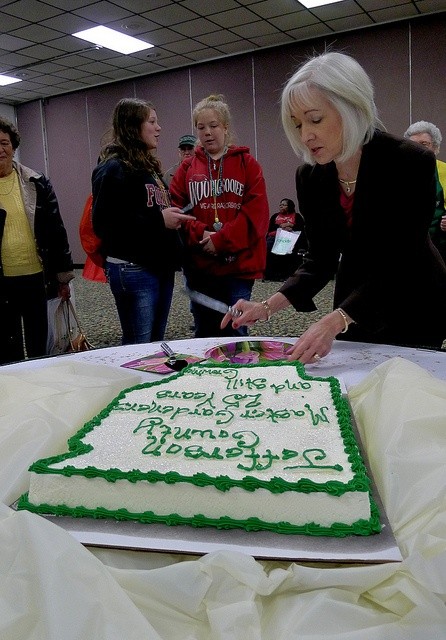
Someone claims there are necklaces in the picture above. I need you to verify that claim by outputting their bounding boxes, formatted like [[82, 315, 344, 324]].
[[203, 146, 229, 197], [336, 175, 356, 192]]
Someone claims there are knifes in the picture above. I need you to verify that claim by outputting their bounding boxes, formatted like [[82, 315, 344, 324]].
[[181, 286, 242, 317]]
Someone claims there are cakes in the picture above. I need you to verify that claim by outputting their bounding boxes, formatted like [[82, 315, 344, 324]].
[[17, 360, 388, 536]]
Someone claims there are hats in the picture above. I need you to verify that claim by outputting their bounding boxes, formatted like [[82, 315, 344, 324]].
[[178, 135, 197, 147]]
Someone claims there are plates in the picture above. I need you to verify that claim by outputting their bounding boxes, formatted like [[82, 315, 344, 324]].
[[204, 340, 305, 365]]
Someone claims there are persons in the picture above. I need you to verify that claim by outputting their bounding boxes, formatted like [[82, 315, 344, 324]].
[[0, 118, 76, 365], [400, 118, 446, 231], [220, 53, 443, 364], [170, 94, 272, 338], [92, 99, 196, 346], [265, 196, 305, 281], [164, 135, 198, 188]]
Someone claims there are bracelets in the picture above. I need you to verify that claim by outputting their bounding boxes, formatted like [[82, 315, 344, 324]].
[[258, 301, 272, 323], [337, 309, 348, 333]]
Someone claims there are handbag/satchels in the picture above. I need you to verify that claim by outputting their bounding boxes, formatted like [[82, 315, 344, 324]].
[[61, 299, 95, 353]]
